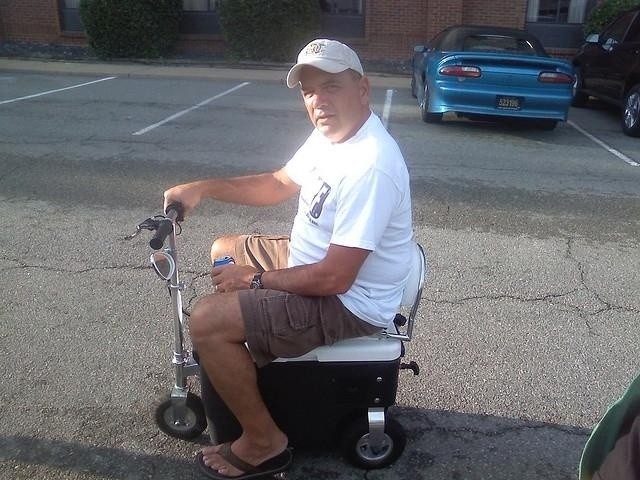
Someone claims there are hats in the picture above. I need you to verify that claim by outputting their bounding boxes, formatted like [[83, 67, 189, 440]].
[[286, 37, 365, 88]]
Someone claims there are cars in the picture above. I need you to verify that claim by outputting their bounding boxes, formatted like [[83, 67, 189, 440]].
[[571, 5, 640, 137], [410, 24, 576, 132]]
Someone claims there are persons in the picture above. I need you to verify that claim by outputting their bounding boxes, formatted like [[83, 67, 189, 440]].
[[164, 36, 410, 480]]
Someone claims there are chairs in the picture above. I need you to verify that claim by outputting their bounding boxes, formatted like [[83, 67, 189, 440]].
[[243, 241, 427, 408]]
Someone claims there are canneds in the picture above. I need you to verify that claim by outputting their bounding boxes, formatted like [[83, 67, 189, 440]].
[[213, 256, 236, 267]]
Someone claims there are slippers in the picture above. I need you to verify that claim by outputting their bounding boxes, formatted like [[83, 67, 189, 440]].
[[196, 439, 294, 480]]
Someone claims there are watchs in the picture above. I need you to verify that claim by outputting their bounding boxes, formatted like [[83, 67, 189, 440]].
[[250, 270, 263, 290]]
